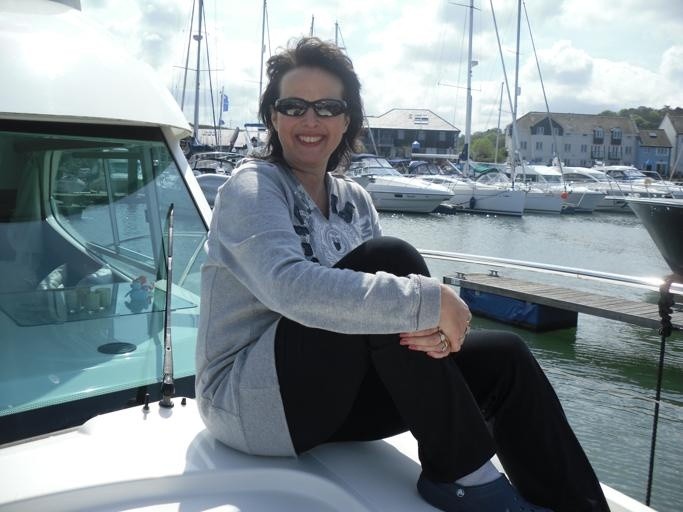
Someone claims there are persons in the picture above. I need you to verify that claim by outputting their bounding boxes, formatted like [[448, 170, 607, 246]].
[[192, 32, 614, 512]]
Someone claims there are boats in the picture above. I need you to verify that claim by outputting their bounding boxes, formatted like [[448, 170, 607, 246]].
[[0, 0, 664, 512], [603, 160, 682, 288]]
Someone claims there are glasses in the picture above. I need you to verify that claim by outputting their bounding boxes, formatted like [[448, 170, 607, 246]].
[[272, 97, 350, 118]]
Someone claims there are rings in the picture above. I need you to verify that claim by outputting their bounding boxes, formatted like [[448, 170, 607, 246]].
[[441, 342, 448, 350], [464, 325, 470, 335], [466, 311, 473, 323], [438, 332, 446, 341]]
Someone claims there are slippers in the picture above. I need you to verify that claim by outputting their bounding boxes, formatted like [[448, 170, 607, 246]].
[[414, 461, 556, 512]]
